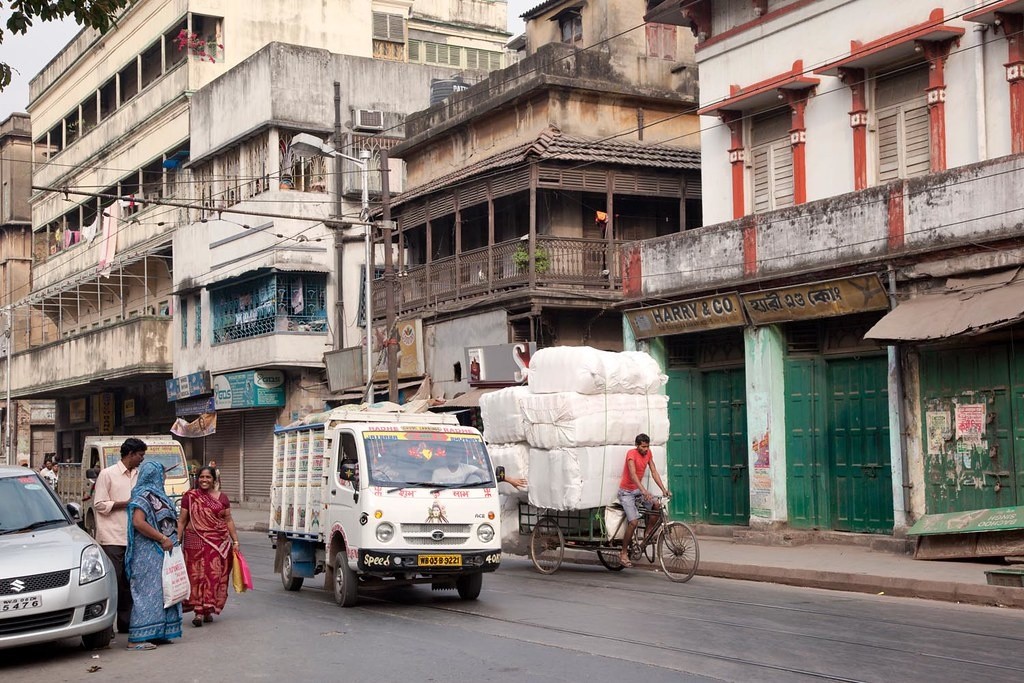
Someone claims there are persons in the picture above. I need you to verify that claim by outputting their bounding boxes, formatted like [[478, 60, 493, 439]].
[[432, 448, 529, 491], [617, 434, 670, 567], [94, 461, 101, 474], [177, 467, 239, 626], [94, 438, 147, 633], [125, 462, 183, 651], [40, 460, 58, 485], [355, 444, 393, 481], [210, 461, 221, 491]]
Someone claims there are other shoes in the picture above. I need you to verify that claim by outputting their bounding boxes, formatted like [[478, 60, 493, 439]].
[[192, 617, 202, 627], [111, 631, 115, 639], [204, 616, 213, 622]]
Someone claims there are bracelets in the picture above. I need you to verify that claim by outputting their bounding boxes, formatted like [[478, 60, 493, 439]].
[[160, 535, 166, 543], [233, 541, 238, 544]]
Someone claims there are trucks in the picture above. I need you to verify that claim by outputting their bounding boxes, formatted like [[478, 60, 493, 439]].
[[57, 435, 199, 539], [266, 411, 507, 608]]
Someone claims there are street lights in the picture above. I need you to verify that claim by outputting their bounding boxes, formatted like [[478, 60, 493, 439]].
[[289, 132, 374, 407]]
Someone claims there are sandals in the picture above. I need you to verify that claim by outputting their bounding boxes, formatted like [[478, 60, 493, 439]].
[[618, 556, 634, 568]]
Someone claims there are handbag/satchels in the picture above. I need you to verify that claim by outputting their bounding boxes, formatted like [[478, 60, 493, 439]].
[[161, 545, 191, 608], [232, 549, 254, 594]]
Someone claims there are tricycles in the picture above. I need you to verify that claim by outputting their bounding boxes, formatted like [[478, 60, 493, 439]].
[[516, 492, 700, 583]]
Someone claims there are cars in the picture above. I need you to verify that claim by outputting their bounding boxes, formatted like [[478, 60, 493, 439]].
[[0, 464, 119, 651]]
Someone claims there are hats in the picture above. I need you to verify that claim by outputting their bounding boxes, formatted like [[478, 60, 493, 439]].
[[21, 462, 28, 466]]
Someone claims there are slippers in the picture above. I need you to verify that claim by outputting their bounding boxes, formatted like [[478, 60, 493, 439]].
[[156, 639, 174, 644], [126, 643, 154, 650]]
